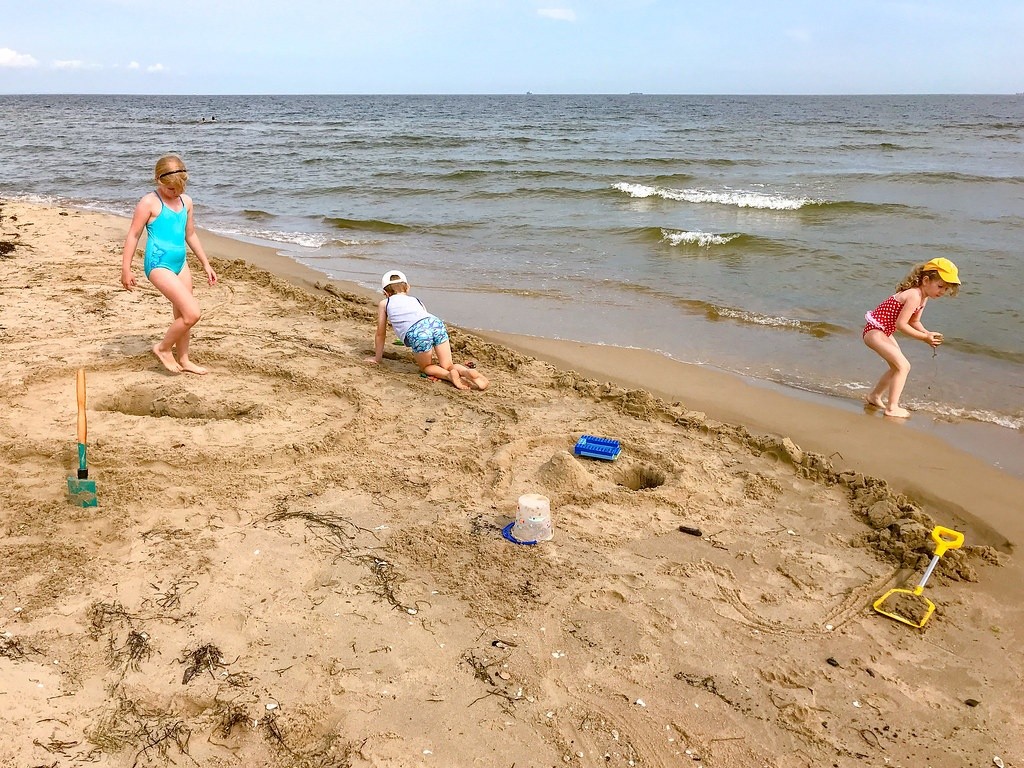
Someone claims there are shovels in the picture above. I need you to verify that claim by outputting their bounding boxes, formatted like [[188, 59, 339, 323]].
[[66, 368, 98, 508], [873, 525, 964, 628]]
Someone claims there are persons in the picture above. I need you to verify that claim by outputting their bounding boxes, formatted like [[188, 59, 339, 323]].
[[363, 269, 490, 390], [121, 156, 218, 375], [863, 257, 962, 417]]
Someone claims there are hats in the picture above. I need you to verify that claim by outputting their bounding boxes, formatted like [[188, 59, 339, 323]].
[[382, 270, 408, 289], [922, 257, 961, 285]]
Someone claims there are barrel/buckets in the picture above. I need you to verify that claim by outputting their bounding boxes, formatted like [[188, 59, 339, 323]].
[[502, 494, 554, 544]]
